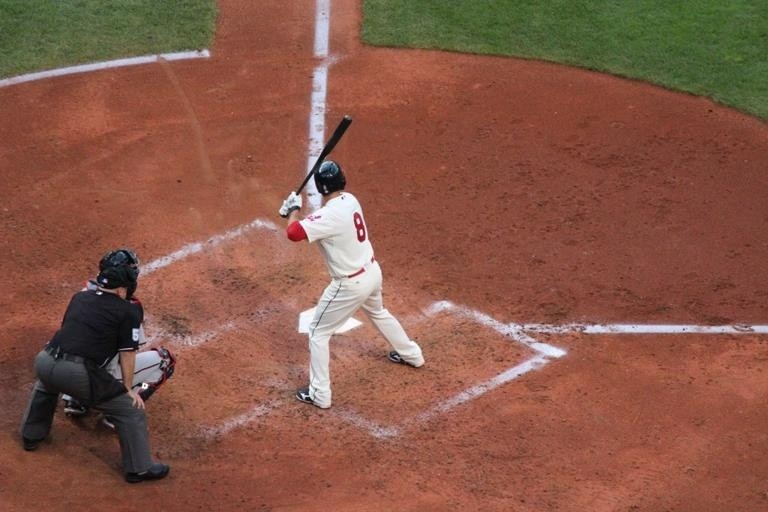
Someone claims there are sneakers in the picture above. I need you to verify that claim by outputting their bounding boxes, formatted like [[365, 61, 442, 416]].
[[64, 402, 86, 414], [389, 351, 415, 368], [102, 417, 115, 430], [127, 464, 169, 482], [23, 437, 45, 450], [296, 390, 314, 405]]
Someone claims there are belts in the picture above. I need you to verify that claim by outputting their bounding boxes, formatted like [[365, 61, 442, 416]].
[[45, 346, 76, 361], [349, 257, 374, 278]]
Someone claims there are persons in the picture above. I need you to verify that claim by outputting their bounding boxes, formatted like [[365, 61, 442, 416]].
[[277, 159, 425, 412], [59, 249, 178, 434], [22, 263, 173, 484]]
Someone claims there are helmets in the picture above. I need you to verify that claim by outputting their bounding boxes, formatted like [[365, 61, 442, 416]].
[[315, 161, 345, 195], [96, 249, 138, 300]]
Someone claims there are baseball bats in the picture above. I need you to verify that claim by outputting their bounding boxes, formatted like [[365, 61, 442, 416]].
[[279, 115, 352, 218]]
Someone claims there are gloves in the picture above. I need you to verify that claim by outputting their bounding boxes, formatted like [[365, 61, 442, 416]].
[[278, 191, 302, 218]]
